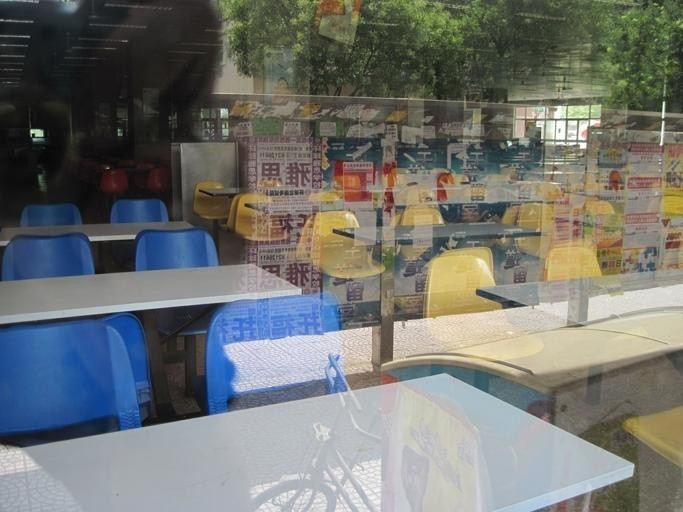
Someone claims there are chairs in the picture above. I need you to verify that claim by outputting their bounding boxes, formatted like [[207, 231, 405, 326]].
[[0, 166, 681, 466], [63, 148, 172, 204]]
